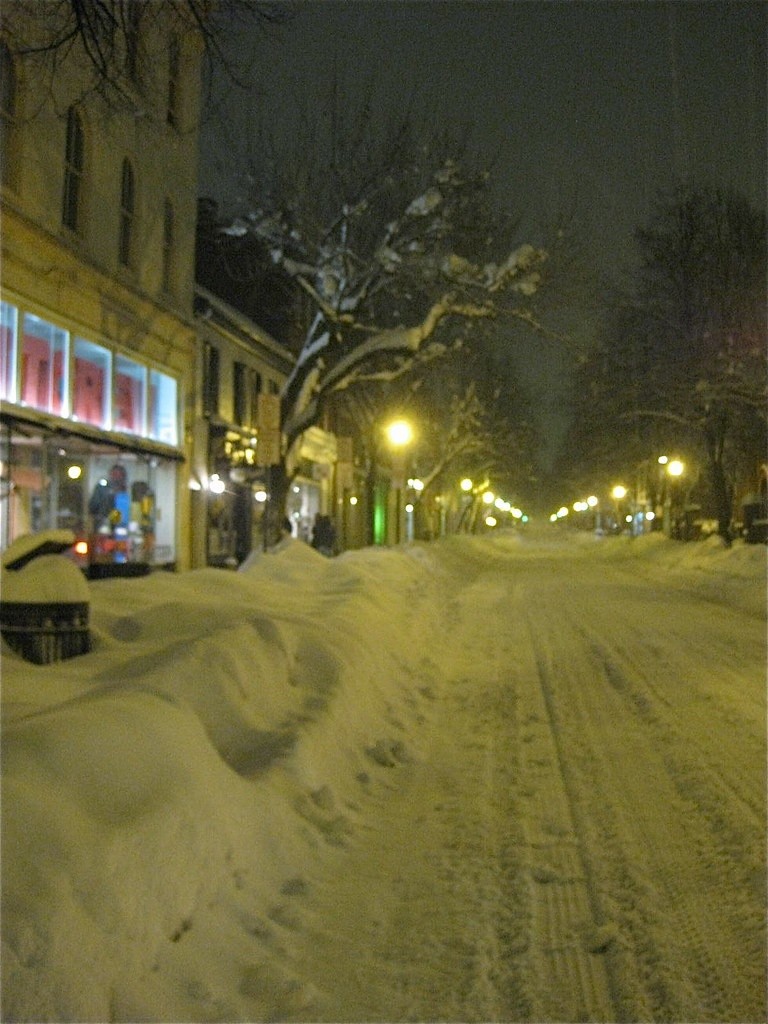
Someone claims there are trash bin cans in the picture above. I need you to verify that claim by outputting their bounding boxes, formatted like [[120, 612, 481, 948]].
[[1, 540, 92, 664]]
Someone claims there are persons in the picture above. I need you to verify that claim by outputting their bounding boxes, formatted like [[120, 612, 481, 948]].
[[311, 513, 341, 556]]
